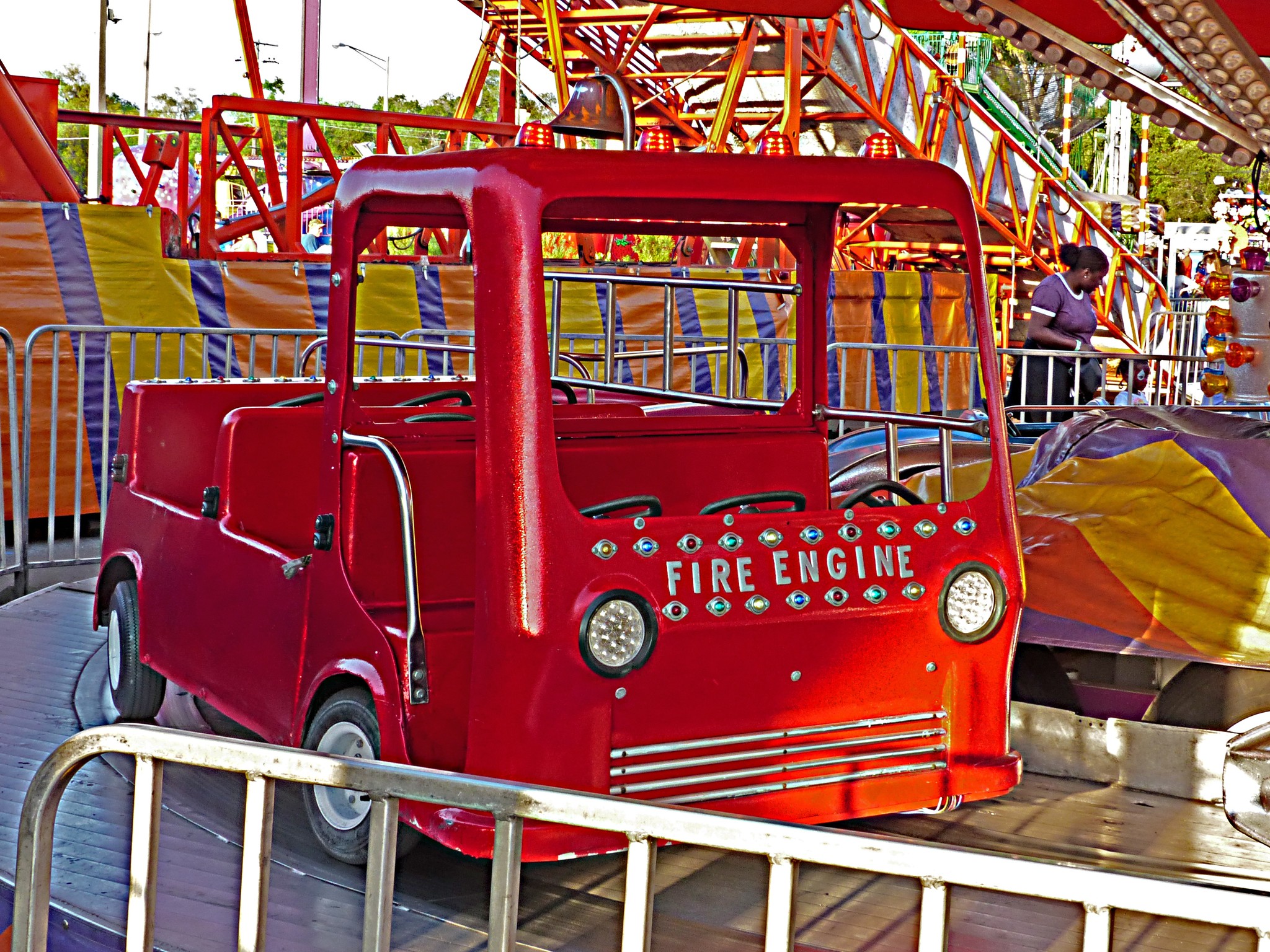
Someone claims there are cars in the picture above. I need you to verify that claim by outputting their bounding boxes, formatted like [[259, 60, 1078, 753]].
[[86, 137, 1028, 880]]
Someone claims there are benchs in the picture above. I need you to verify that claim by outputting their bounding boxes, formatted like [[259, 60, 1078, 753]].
[[124, 372, 828, 765]]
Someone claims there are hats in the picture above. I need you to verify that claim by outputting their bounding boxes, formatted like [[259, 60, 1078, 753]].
[[215, 210, 221, 217], [308, 219, 326, 228]]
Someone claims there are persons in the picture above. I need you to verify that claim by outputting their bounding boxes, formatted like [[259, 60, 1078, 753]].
[[214, 211, 233, 254], [1114, 359, 1151, 406], [302, 218, 327, 255], [1003, 242, 1110, 423], [1139, 245, 1232, 312]]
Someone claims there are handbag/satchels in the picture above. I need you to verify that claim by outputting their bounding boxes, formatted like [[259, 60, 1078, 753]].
[[1068, 357, 1102, 398]]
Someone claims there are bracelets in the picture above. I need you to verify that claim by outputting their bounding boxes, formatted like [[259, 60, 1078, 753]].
[[1074, 339, 1081, 351]]
